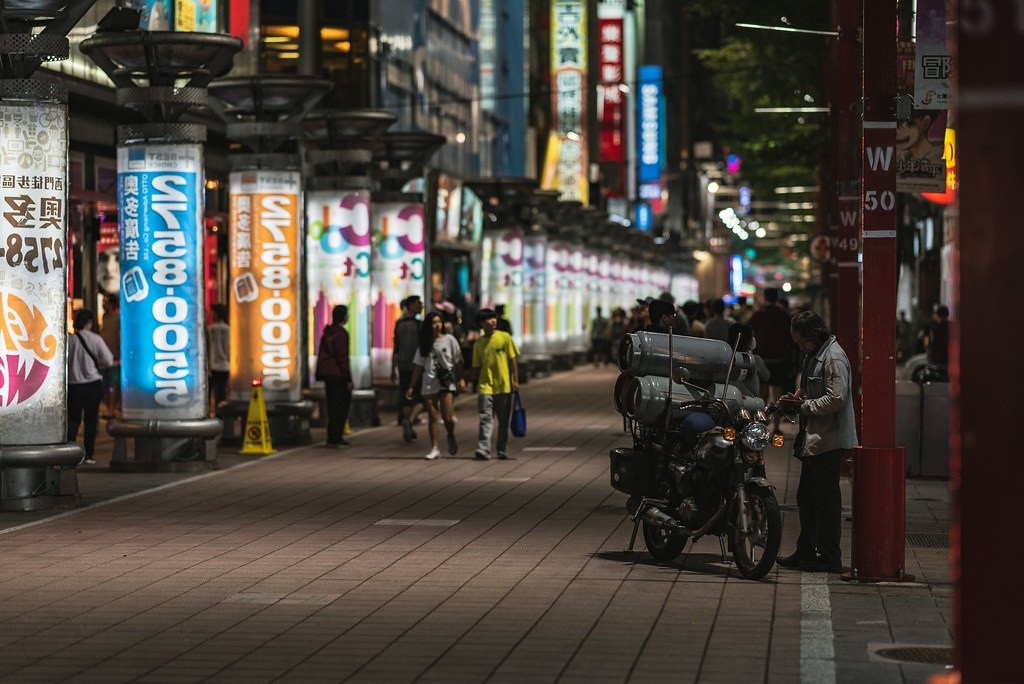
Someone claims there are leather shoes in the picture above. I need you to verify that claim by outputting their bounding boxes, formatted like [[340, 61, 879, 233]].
[[776, 552, 817, 568], [799, 556, 840, 573]]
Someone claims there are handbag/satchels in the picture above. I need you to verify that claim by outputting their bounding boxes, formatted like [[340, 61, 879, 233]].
[[435, 367, 457, 392], [510, 391, 527, 437]]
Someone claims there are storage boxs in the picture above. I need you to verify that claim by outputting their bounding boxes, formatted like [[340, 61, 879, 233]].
[[607, 447, 648, 496]]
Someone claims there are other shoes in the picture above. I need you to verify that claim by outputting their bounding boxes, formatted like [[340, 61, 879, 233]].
[[327, 439, 351, 448], [448, 434, 457, 455], [85, 458, 96, 465], [426, 448, 441, 461], [475, 449, 491, 459], [403, 419, 412, 442], [498, 450, 508, 460]]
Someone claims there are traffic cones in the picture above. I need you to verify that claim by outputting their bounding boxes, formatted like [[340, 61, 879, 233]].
[[238, 379, 278, 455]]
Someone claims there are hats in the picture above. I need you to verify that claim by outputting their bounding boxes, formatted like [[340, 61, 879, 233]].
[[436, 301, 455, 314]]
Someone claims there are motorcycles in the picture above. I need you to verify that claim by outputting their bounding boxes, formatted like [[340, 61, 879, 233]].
[[610, 326, 805, 579]]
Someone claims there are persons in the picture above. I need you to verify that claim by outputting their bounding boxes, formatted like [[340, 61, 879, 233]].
[[747, 288, 805, 435], [775, 311, 858, 573], [897, 310, 910, 335], [69, 309, 113, 464], [592, 296, 753, 369], [926, 307, 950, 382], [207, 303, 230, 419], [390, 296, 521, 459], [98, 294, 120, 420], [315, 305, 354, 448]]
